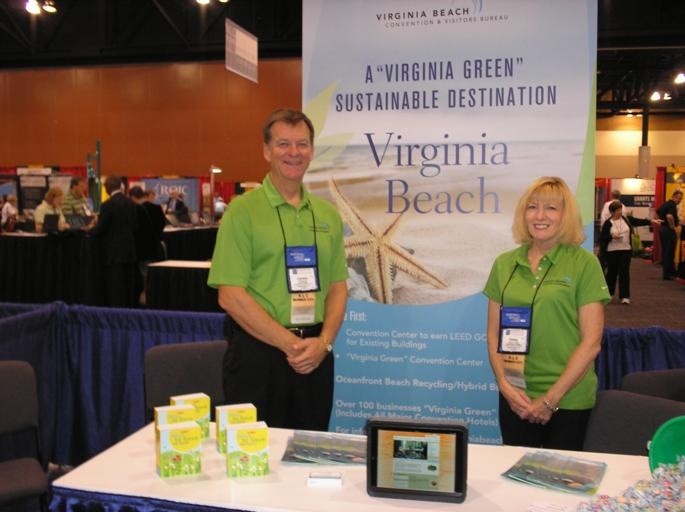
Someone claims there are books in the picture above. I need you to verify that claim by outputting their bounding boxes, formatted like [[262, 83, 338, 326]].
[[511, 449, 607, 498], [499, 452, 566, 494]]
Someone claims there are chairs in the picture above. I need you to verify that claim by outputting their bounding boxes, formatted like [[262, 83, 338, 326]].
[[623, 368, 685, 404], [580, 388, 684, 457], [142, 340, 228, 430], [0, 360, 49, 511]]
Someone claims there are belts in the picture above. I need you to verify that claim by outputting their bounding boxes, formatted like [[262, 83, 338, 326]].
[[285, 326, 323, 340]]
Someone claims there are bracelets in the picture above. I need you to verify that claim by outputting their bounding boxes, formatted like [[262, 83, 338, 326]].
[[542, 395, 560, 413]]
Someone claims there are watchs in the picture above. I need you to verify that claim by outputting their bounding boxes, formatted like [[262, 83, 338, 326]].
[[316, 332, 336, 354]]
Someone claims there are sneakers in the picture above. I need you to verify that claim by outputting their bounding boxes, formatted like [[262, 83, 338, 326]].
[[621, 298, 630, 305]]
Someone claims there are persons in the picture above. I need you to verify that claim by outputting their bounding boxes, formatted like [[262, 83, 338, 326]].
[[124, 185, 166, 306], [142, 190, 157, 204], [202, 106, 350, 434], [600, 197, 666, 307], [597, 188, 630, 281], [210, 191, 225, 204], [90, 175, 140, 308], [0, 193, 21, 228], [479, 172, 618, 452], [212, 197, 230, 221], [655, 190, 685, 281], [59, 174, 99, 231], [164, 184, 191, 227], [33, 186, 72, 235]]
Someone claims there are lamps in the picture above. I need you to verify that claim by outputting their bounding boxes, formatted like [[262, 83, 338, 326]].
[[24, 0, 57, 15], [649, 69, 685, 102]]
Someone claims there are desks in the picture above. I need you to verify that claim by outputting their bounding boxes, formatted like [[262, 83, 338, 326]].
[[0, 223, 220, 312], [52, 419, 648, 512]]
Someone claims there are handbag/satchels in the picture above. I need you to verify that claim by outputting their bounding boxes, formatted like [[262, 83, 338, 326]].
[[629, 233, 641, 257]]
